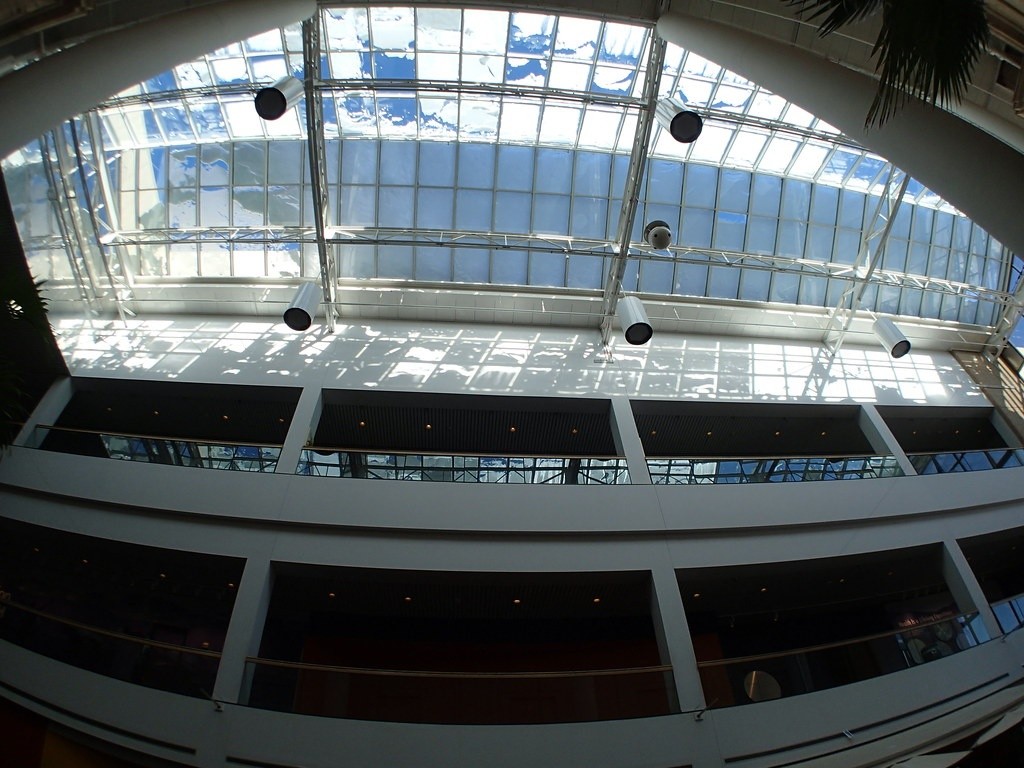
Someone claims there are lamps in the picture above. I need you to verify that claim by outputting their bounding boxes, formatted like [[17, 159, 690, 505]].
[[616, 279, 653, 345], [857, 298, 912, 358], [654, 97, 703, 143], [255, 76, 312, 120], [283, 264, 327, 330], [644, 220, 672, 250]]
